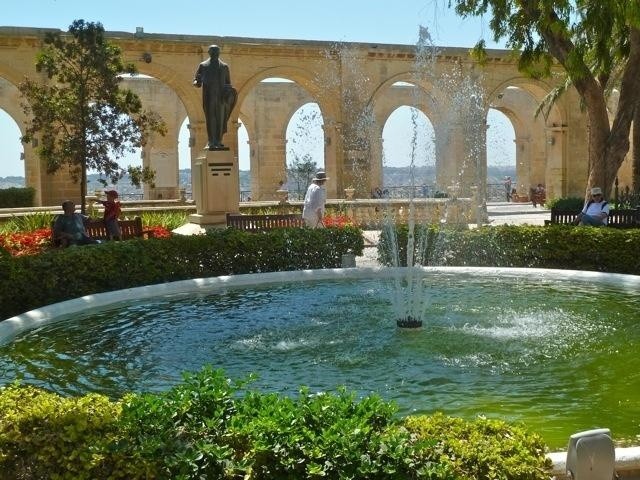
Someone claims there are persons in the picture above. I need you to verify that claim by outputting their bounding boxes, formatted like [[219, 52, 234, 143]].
[[89, 190, 122, 241], [279, 181, 287, 190], [504, 176, 511, 201], [193, 43, 237, 151], [303, 171, 330, 229], [571, 188, 609, 228], [54, 199, 99, 247], [536, 184, 544, 194]]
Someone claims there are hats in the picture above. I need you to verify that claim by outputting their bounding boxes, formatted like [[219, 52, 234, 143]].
[[313, 172, 328, 180], [105, 190, 118, 198], [590, 187, 603, 196]]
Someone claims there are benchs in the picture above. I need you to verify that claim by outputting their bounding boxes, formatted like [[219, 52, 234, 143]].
[[225, 212, 305, 234], [544, 208, 639, 230], [51, 218, 156, 251]]
